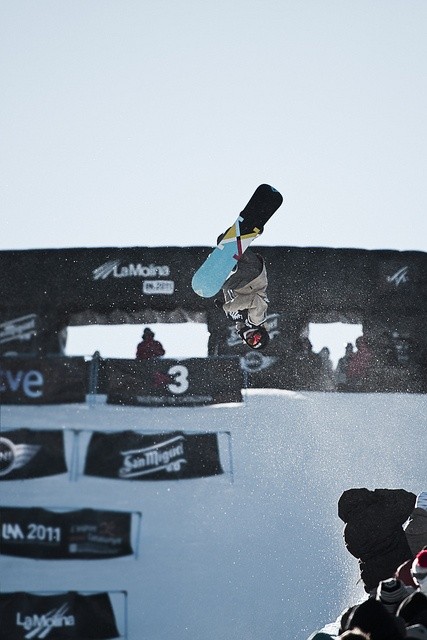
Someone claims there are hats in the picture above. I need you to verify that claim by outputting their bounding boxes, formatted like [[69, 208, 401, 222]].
[[142, 328, 154, 336], [417, 492, 427, 511], [410, 550, 427, 586], [375, 578, 410, 613]]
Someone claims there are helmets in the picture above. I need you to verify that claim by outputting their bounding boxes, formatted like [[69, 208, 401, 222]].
[[244, 327, 269, 350]]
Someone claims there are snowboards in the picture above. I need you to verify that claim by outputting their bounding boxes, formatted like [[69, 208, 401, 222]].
[[191, 184, 283, 298]]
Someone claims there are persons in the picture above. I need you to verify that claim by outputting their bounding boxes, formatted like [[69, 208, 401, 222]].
[[300, 332, 427, 392], [308, 487, 427, 639], [136, 327, 166, 362], [212, 226, 271, 350]]
